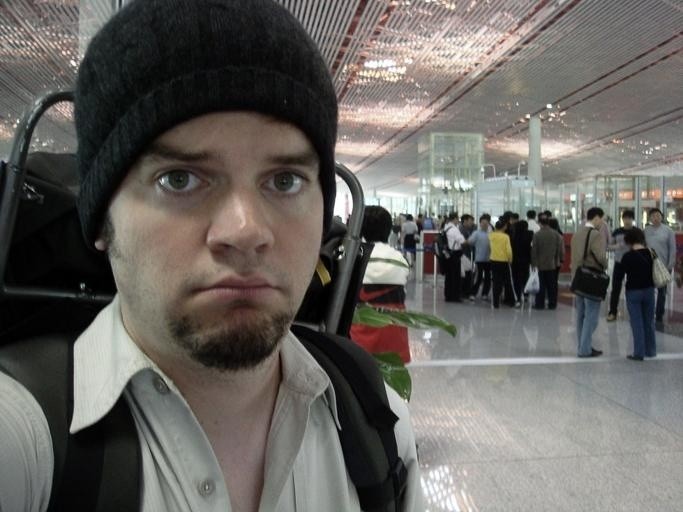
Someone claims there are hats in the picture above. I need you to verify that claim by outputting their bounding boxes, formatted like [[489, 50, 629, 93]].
[[74, 0, 335, 247]]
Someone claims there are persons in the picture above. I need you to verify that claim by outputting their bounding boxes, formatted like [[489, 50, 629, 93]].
[[439, 211, 564, 310], [570, 206, 676, 361], [388, 213, 443, 267], [0, 0, 427, 512]]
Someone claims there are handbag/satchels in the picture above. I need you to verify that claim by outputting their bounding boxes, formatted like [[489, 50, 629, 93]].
[[570, 266, 609, 301], [652, 258, 671, 287]]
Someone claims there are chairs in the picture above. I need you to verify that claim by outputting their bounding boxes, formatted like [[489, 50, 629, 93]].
[[348, 285, 409, 367]]
